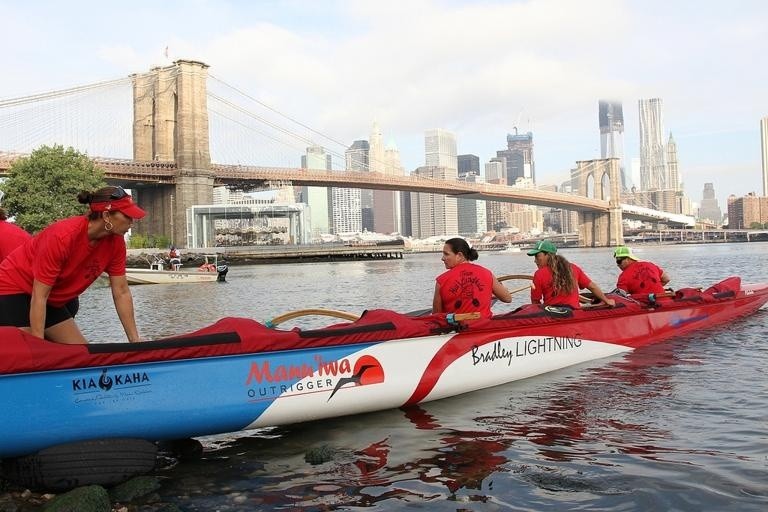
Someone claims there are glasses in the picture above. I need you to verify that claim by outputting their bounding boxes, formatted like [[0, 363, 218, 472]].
[[617, 257, 626, 264]]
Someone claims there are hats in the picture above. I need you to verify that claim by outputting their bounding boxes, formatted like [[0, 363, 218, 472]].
[[613, 247, 639, 261], [527, 240, 557, 256], [90, 195, 146, 219]]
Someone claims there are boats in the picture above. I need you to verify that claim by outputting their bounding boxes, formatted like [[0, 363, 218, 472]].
[[98, 265, 229, 285], [0, 283, 767, 459]]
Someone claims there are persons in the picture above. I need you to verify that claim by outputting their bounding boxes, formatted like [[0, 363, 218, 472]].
[[527, 240, 617, 308], [612, 245, 671, 301], [0, 186, 149, 346], [169, 245, 182, 270], [432, 238, 512, 323]]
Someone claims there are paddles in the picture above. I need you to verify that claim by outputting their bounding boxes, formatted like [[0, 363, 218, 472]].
[[404, 284, 532, 317]]
